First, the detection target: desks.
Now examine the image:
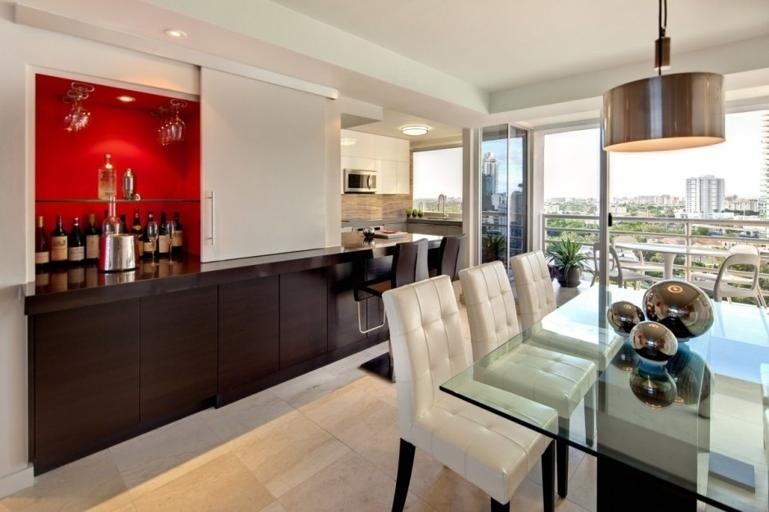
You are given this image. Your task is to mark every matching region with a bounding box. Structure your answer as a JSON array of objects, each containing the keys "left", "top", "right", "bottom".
[{"left": 438, "top": 277, "right": 768, "bottom": 511}]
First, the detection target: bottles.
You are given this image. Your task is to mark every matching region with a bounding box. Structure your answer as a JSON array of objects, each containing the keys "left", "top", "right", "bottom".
[
  {"left": 96, "top": 153, "right": 116, "bottom": 200},
  {"left": 99, "top": 195, "right": 119, "bottom": 234},
  {"left": 69, "top": 268, "right": 85, "bottom": 287},
  {"left": 87, "top": 267, "right": 98, "bottom": 286},
  {"left": 159, "top": 213, "right": 170, "bottom": 258},
  {"left": 172, "top": 261, "right": 183, "bottom": 274},
  {"left": 144, "top": 211, "right": 159, "bottom": 259},
  {"left": 158, "top": 261, "right": 170, "bottom": 277},
  {"left": 35, "top": 271, "right": 50, "bottom": 293},
  {"left": 140, "top": 261, "right": 156, "bottom": 278},
  {"left": 51, "top": 269, "right": 68, "bottom": 291},
  {"left": 170, "top": 211, "right": 184, "bottom": 259},
  {"left": 51, "top": 216, "right": 68, "bottom": 268},
  {"left": 68, "top": 217, "right": 86, "bottom": 266},
  {"left": 85, "top": 213, "right": 100, "bottom": 265},
  {"left": 132, "top": 211, "right": 144, "bottom": 260},
  {"left": 120, "top": 213, "right": 127, "bottom": 233},
  {"left": 124, "top": 169, "right": 133, "bottom": 199},
  {"left": 34, "top": 215, "right": 50, "bottom": 269}
]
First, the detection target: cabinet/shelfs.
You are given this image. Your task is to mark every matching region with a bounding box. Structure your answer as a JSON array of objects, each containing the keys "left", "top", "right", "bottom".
[
  {"left": 379, "top": 136, "right": 410, "bottom": 195},
  {"left": 339, "top": 129, "right": 380, "bottom": 162}
]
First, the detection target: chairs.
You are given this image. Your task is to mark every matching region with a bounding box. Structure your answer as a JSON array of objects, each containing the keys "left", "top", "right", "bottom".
[
  {"left": 587, "top": 222, "right": 768, "bottom": 310},
  {"left": 352, "top": 234, "right": 471, "bottom": 382},
  {"left": 510, "top": 248, "right": 634, "bottom": 446},
  {"left": 457, "top": 260, "right": 593, "bottom": 501},
  {"left": 379, "top": 272, "right": 559, "bottom": 512}
]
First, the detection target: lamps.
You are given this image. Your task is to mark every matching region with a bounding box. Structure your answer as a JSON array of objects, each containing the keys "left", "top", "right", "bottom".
[
  {"left": 599, "top": 0, "right": 726, "bottom": 155},
  {"left": 401, "top": 127, "right": 429, "bottom": 138}
]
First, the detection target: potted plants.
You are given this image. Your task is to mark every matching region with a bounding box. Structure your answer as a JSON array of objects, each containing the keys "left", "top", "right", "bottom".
[
  {"left": 548, "top": 238, "right": 585, "bottom": 288},
  {"left": 404, "top": 209, "right": 424, "bottom": 219}
]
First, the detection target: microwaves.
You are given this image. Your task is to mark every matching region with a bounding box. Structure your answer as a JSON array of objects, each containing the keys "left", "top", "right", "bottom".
[{"left": 343, "top": 169, "right": 376, "bottom": 193}]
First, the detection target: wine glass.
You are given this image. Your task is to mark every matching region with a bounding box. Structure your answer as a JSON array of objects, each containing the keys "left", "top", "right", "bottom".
[
  {"left": 64, "top": 94, "right": 91, "bottom": 132},
  {"left": 156, "top": 104, "right": 186, "bottom": 147}
]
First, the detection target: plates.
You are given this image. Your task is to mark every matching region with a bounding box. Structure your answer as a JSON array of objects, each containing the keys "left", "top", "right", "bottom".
[{"left": 384, "top": 230, "right": 399, "bottom": 234}]
